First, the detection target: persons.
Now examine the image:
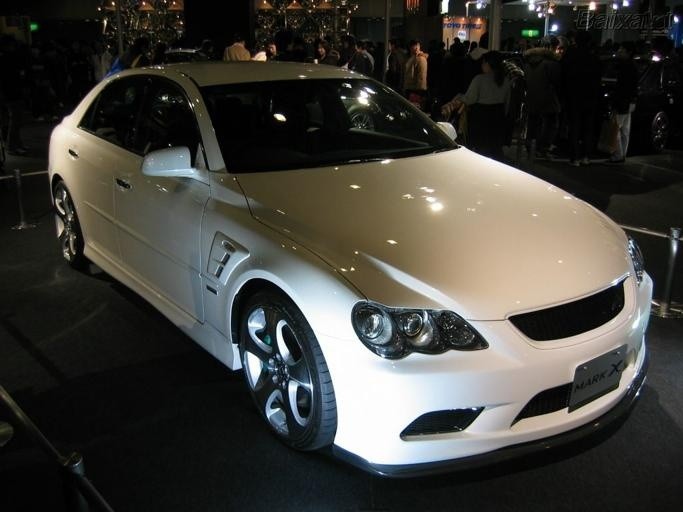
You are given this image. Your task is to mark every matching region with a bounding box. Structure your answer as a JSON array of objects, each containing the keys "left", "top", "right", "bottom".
[{"left": 1, "top": 24, "right": 642, "bottom": 167}]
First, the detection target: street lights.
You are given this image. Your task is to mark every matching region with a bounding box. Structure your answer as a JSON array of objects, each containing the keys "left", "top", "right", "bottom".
[
  {"left": 529, "top": 0, "right": 558, "bottom": 37},
  {"left": 466, "top": 0, "right": 488, "bottom": 41}
]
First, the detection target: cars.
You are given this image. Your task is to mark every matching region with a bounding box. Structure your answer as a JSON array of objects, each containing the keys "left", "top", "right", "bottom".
[
  {"left": 594, "top": 55, "right": 682, "bottom": 155},
  {"left": 48, "top": 60, "right": 655, "bottom": 480}
]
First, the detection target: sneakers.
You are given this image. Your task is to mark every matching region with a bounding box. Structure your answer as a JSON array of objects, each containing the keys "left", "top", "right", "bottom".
[{"left": 531, "top": 152, "right": 628, "bottom": 168}]
